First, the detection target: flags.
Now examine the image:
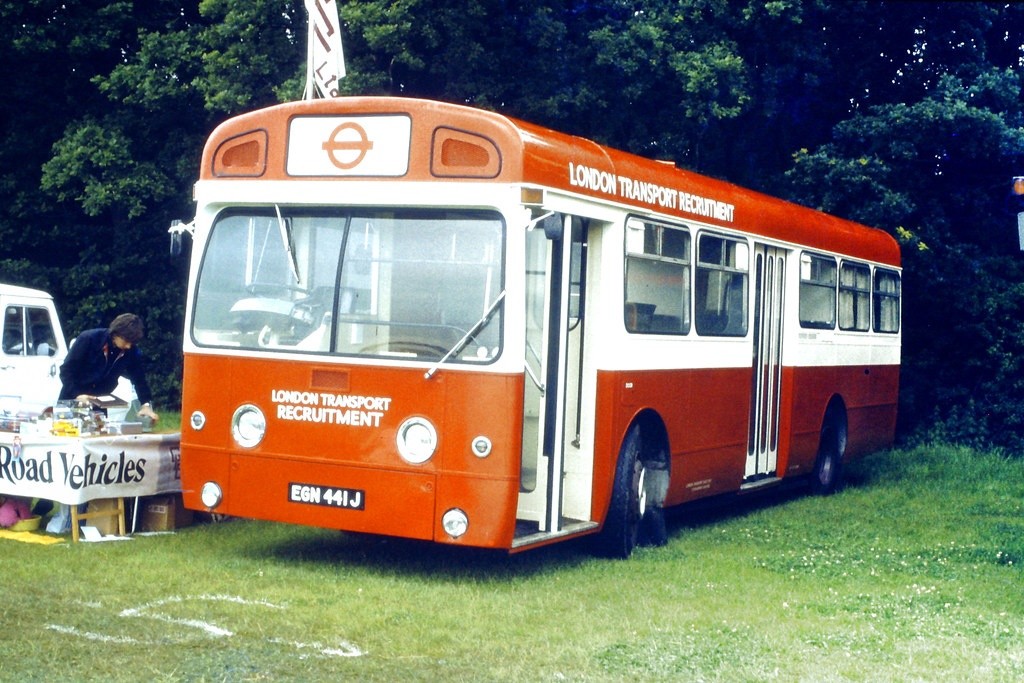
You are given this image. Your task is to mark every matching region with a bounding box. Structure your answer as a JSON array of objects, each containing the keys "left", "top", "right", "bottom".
[{"left": 304, "top": 0, "right": 347, "bottom": 101}]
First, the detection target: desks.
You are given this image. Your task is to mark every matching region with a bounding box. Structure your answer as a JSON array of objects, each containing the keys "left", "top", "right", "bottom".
[{"left": 0, "top": 431, "right": 184, "bottom": 543}]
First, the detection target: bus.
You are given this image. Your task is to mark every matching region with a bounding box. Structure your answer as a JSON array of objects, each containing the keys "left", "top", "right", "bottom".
[{"left": 170, "top": 95, "right": 903, "bottom": 560}]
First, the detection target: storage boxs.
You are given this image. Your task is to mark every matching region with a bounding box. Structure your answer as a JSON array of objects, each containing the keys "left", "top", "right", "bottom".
[{"left": 140, "top": 493, "right": 195, "bottom": 531}]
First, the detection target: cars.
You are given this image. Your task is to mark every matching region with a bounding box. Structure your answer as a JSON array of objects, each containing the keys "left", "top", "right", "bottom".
[{"left": 1, "top": 282, "right": 78, "bottom": 414}]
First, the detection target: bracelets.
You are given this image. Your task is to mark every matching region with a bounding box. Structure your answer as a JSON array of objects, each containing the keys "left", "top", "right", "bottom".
[{"left": 141, "top": 402, "right": 152, "bottom": 407}]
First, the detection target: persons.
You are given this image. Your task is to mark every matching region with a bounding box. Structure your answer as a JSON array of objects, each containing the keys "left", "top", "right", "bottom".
[{"left": 31, "top": 314, "right": 158, "bottom": 516}]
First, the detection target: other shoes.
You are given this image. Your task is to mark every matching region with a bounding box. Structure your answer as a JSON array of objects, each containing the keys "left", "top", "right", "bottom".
[{"left": 32, "top": 500, "right": 55, "bottom": 517}]
